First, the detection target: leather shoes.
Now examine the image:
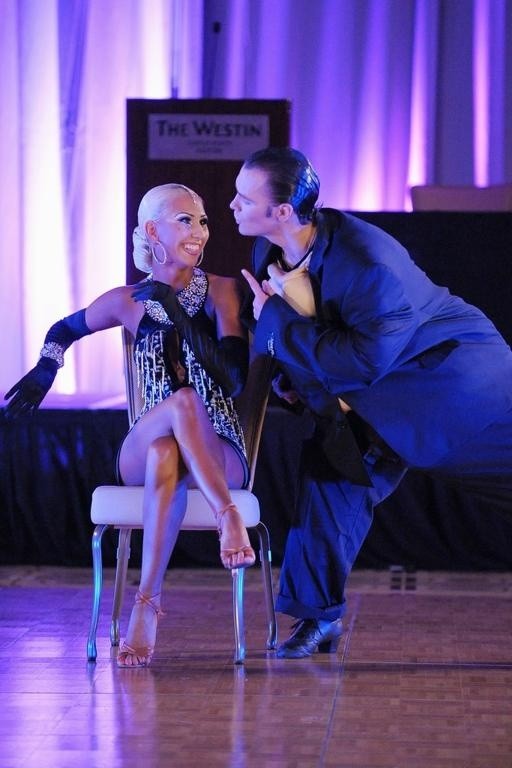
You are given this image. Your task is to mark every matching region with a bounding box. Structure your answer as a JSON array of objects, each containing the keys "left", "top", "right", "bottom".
[{"left": 276, "top": 612, "right": 344, "bottom": 660}]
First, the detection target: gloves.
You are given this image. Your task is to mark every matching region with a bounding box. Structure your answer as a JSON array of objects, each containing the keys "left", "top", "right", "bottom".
[
  {"left": 129, "top": 278, "right": 250, "bottom": 396},
  {"left": 5, "top": 308, "right": 92, "bottom": 417}
]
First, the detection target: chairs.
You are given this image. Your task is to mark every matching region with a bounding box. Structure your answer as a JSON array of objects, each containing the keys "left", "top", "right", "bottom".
[{"left": 85, "top": 324, "right": 279, "bottom": 665}]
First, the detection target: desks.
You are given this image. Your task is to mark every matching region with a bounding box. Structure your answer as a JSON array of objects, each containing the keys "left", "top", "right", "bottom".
[{"left": 1, "top": 407, "right": 294, "bottom": 567}]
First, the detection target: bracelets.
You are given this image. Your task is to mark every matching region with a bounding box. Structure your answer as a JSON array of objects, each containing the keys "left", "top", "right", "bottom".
[{"left": 39, "top": 343, "right": 65, "bottom": 367}]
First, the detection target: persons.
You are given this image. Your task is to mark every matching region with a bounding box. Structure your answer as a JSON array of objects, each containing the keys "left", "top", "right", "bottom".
[
  {"left": 6, "top": 183, "right": 255, "bottom": 669},
  {"left": 231, "top": 147, "right": 512, "bottom": 659}
]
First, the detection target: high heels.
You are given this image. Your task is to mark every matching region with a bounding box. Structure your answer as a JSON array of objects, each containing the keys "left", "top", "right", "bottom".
[
  {"left": 116, "top": 588, "right": 165, "bottom": 669},
  {"left": 214, "top": 502, "right": 256, "bottom": 576}
]
